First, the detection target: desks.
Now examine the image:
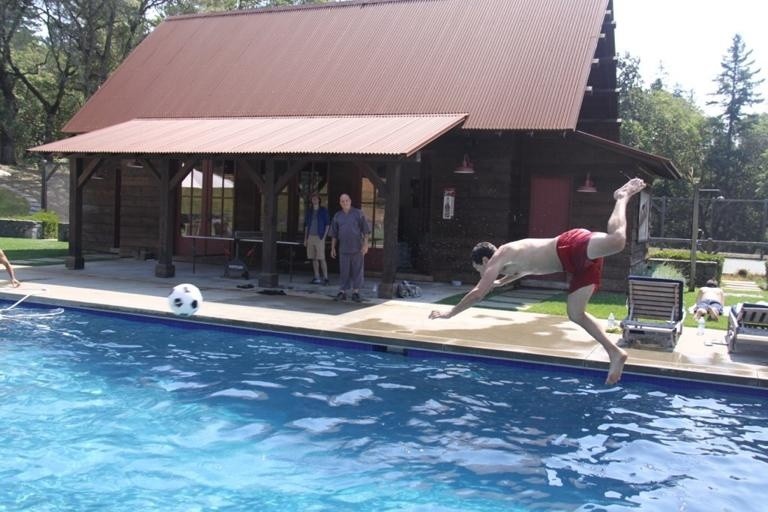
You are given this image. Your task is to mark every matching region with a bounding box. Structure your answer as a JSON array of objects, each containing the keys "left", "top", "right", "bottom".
[{"left": 184, "top": 231, "right": 304, "bottom": 283}]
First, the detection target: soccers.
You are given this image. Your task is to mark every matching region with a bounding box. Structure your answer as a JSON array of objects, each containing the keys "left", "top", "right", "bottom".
[{"left": 169, "top": 283, "right": 203, "bottom": 318}]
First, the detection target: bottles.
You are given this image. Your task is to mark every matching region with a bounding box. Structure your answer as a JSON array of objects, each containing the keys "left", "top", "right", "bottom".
[
  {"left": 607, "top": 313, "right": 616, "bottom": 329},
  {"left": 697, "top": 317, "right": 705, "bottom": 335}
]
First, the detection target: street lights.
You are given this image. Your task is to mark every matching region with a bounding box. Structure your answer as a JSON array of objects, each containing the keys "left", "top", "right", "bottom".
[{"left": 687, "top": 185, "right": 726, "bottom": 295}]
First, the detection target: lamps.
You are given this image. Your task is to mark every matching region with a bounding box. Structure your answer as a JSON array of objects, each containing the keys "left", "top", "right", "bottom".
[
  {"left": 576, "top": 171, "right": 597, "bottom": 193},
  {"left": 127, "top": 159, "right": 144, "bottom": 168},
  {"left": 453, "top": 154, "right": 474, "bottom": 174}
]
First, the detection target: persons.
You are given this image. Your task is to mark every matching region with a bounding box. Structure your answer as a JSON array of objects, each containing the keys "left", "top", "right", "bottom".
[
  {"left": 0, "top": 249, "right": 20, "bottom": 287},
  {"left": 693, "top": 279, "right": 723, "bottom": 321},
  {"left": 327, "top": 194, "right": 372, "bottom": 302},
  {"left": 304, "top": 192, "right": 330, "bottom": 286},
  {"left": 429, "top": 178, "right": 646, "bottom": 385}
]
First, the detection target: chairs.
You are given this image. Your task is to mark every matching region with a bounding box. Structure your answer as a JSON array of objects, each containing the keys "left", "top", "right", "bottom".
[
  {"left": 727, "top": 303, "right": 768, "bottom": 354},
  {"left": 620, "top": 275, "right": 686, "bottom": 347}
]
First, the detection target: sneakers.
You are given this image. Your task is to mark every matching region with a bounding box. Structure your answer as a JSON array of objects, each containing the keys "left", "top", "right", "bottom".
[
  {"left": 309, "top": 278, "right": 320, "bottom": 284},
  {"left": 334, "top": 292, "right": 347, "bottom": 300},
  {"left": 323, "top": 278, "right": 329, "bottom": 286},
  {"left": 351, "top": 293, "right": 361, "bottom": 303}
]
